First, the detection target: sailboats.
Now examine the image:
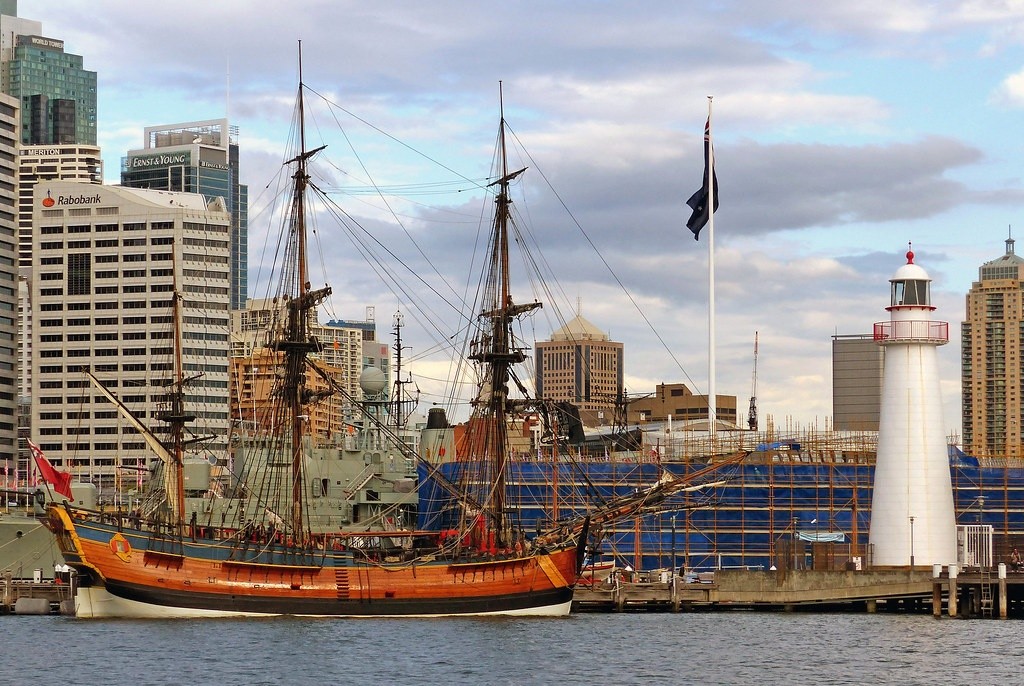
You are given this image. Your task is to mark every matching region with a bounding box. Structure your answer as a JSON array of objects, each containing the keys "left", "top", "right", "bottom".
[{"left": 23, "top": 40, "right": 752, "bottom": 618}]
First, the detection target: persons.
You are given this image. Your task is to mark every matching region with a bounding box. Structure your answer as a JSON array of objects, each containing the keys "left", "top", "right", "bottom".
[
  {"left": 128, "top": 508, "right": 141, "bottom": 530},
  {"left": 268, "top": 521, "right": 275, "bottom": 544},
  {"left": 255, "top": 521, "right": 266, "bottom": 542},
  {"left": 1010, "top": 548, "right": 1021, "bottom": 575},
  {"left": 245, "top": 519, "right": 254, "bottom": 540}
]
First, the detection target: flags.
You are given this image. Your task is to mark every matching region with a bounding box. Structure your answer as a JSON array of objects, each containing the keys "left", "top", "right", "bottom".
[
  {"left": 138, "top": 460, "right": 144, "bottom": 474},
  {"left": 5, "top": 462, "right": 8, "bottom": 483},
  {"left": 14, "top": 468, "right": 16, "bottom": 491},
  {"left": 32, "top": 466, "right": 36, "bottom": 488},
  {"left": 27, "top": 437, "right": 73, "bottom": 500},
  {"left": 138, "top": 476, "right": 142, "bottom": 487},
  {"left": 686, "top": 114, "right": 718, "bottom": 241}
]
